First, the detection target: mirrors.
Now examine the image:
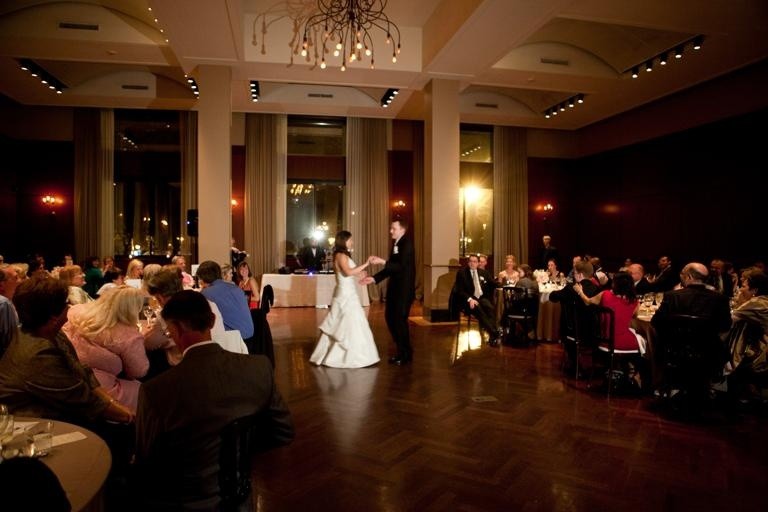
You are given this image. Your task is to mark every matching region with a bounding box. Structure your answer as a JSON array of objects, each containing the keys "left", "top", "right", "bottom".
[{"left": 286, "top": 185, "right": 345, "bottom": 254}]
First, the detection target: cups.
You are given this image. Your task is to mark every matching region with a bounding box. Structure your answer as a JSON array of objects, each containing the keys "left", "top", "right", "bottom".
[
  {"left": 636, "top": 294, "right": 662, "bottom": 315},
  {"left": 0, "top": 405, "right": 52, "bottom": 461}
]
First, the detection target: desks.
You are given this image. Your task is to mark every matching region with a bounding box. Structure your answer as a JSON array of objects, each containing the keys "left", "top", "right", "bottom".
[{"left": 258, "top": 273, "right": 373, "bottom": 312}]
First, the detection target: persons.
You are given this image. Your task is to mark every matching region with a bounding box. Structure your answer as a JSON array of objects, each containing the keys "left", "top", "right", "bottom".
[
  {"left": 447, "top": 253, "right": 767, "bottom": 440},
  {"left": 540, "top": 235, "right": 564, "bottom": 266},
  {"left": 2, "top": 238, "right": 296, "bottom": 512},
  {"left": 291, "top": 237, "right": 327, "bottom": 275},
  {"left": 359, "top": 217, "right": 416, "bottom": 367},
  {"left": 308, "top": 230, "right": 381, "bottom": 369}
]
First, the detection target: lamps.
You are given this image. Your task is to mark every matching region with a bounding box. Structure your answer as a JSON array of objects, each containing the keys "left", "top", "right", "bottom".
[
  {"left": 631, "top": 39, "right": 702, "bottom": 81},
  {"left": 287, "top": 183, "right": 314, "bottom": 206},
  {"left": 251, "top": 1, "right": 404, "bottom": 71},
  {"left": 21, "top": 57, "right": 63, "bottom": 96},
  {"left": 379, "top": 88, "right": 400, "bottom": 110},
  {"left": 41, "top": 193, "right": 65, "bottom": 215},
  {"left": 184, "top": 74, "right": 200, "bottom": 101},
  {"left": 231, "top": 200, "right": 237, "bottom": 220},
  {"left": 540, "top": 203, "right": 553, "bottom": 223},
  {"left": 392, "top": 200, "right": 406, "bottom": 223},
  {"left": 541, "top": 94, "right": 587, "bottom": 121},
  {"left": 248, "top": 80, "right": 261, "bottom": 105}
]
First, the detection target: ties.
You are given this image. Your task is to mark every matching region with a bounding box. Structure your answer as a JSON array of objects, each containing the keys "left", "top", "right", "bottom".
[{"left": 473, "top": 270, "right": 480, "bottom": 299}]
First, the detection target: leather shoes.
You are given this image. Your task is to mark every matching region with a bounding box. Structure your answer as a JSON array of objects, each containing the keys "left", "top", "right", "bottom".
[
  {"left": 490, "top": 338, "right": 497, "bottom": 344},
  {"left": 493, "top": 331, "right": 503, "bottom": 338},
  {"left": 396, "top": 357, "right": 412, "bottom": 365},
  {"left": 390, "top": 355, "right": 402, "bottom": 362}
]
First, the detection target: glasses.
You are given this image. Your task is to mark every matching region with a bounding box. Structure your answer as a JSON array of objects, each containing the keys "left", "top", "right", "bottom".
[
  {"left": 468, "top": 260, "right": 478, "bottom": 264},
  {"left": 162, "top": 320, "right": 177, "bottom": 339},
  {"left": 64, "top": 298, "right": 72, "bottom": 305}
]
